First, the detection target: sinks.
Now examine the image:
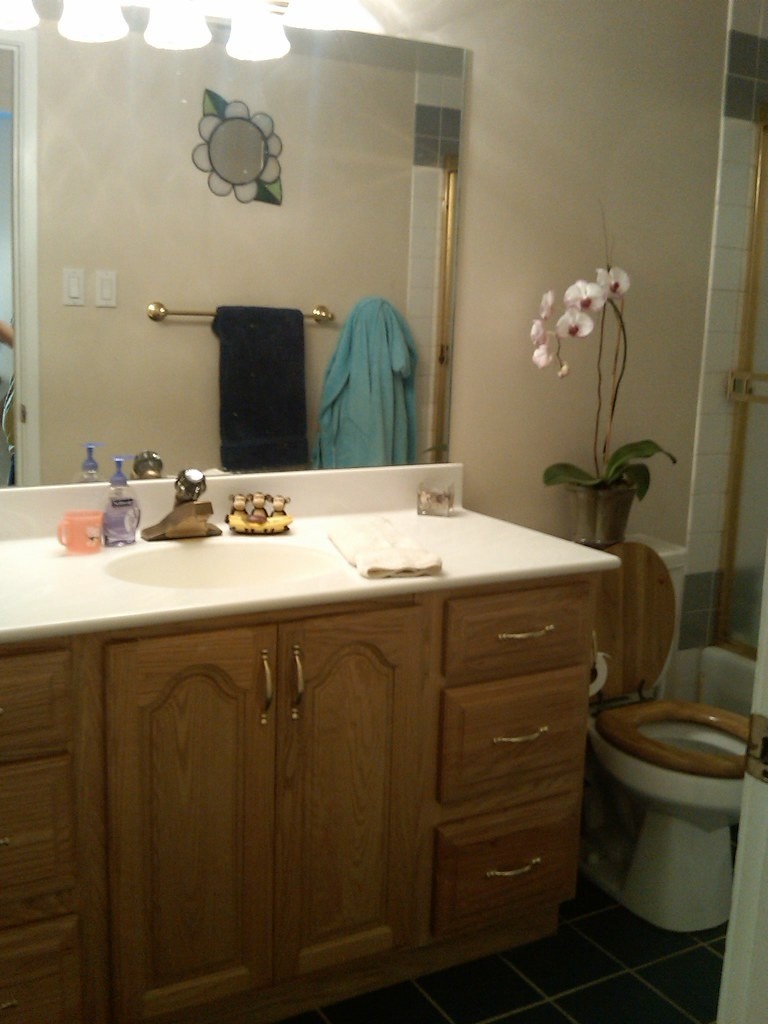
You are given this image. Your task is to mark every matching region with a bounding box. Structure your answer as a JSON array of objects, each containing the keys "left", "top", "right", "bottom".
[{"left": 101, "top": 534, "right": 334, "bottom": 593}]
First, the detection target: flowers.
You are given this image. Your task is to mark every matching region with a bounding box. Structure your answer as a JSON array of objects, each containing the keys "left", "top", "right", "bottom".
[{"left": 522, "top": 268, "right": 679, "bottom": 501}]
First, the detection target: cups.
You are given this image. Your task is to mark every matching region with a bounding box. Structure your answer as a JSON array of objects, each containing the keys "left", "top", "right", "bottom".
[
  {"left": 416, "top": 478, "right": 454, "bottom": 516},
  {"left": 57, "top": 510, "right": 103, "bottom": 554}
]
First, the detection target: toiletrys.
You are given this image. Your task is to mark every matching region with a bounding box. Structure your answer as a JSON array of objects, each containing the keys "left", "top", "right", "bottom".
[
  {"left": 101, "top": 456, "right": 140, "bottom": 547},
  {"left": 74, "top": 444, "right": 105, "bottom": 483}
]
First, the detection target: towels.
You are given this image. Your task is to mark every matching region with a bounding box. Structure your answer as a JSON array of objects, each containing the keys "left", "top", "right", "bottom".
[
  {"left": 327, "top": 514, "right": 443, "bottom": 581},
  {"left": 210, "top": 305, "right": 310, "bottom": 474},
  {"left": 311, "top": 296, "right": 419, "bottom": 472}
]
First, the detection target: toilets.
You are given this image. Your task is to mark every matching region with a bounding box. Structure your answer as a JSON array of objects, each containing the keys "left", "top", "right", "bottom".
[{"left": 569, "top": 530, "right": 750, "bottom": 932}]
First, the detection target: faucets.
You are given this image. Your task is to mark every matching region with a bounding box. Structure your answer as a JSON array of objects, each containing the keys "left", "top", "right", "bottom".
[{"left": 141, "top": 469, "right": 223, "bottom": 541}]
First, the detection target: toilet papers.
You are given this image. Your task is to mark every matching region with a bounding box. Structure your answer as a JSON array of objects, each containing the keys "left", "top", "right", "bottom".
[{"left": 590, "top": 651, "right": 611, "bottom": 697}]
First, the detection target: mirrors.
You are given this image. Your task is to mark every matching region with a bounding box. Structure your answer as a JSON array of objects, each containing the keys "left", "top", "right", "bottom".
[{"left": 0, "top": 1, "right": 469, "bottom": 489}]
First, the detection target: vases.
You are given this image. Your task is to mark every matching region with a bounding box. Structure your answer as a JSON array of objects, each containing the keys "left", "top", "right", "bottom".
[{"left": 563, "top": 483, "right": 632, "bottom": 542}]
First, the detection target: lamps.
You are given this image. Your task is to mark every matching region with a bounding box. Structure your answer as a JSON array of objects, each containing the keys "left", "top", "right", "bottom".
[
  {"left": 58, "top": 1, "right": 128, "bottom": 45},
  {"left": 145, "top": 0, "right": 211, "bottom": 50},
  {"left": 0, "top": 0, "right": 40, "bottom": 32},
  {"left": 226, "top": 1, "right": 292, "bottom": 62}
]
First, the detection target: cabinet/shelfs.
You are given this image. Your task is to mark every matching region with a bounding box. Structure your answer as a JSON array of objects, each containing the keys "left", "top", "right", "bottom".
[
  {"left": 0, "top": 645, "right": 84, "bottom": 1024},
  {"left": 101, "top": 600, "right": 425, "bottom": 1021},
  {"left": 430, "top": 584, "right": 578, "bottom": 946}
]
